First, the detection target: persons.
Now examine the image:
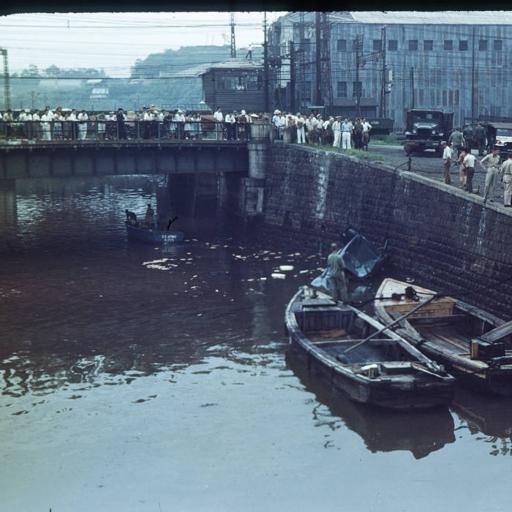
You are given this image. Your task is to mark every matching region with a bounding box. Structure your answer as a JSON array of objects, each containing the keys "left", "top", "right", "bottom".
[
  {"left": 272, "top": 109, "right": 372, "bottom": 152},
  {"left": 461, "top": 149, "right": 481, "bottom": 194},
  {"left": 473, "top": 121, "right": 487, "bottom": 155},
  {"left": 326, "top": 242, "right": 351, "bottom": 305},
  {"left": 145, "top": 202, "right": 154, "bottom": 229},
  {"left": 213, "top": 107, "right": 252, "bottom": 141},
  {"left": 124, "top": 208, "right": 137, "bottom": 226},
  {"left": 498, "top": 153, "right": 512, "bottom": 207},
  {"left": 457, "top": 145, "right": 467, "bottom": 184},
  {"left": 478, "top": 148, "right": 503, "bottom": 204},
  {"left": 462, "top": 122, "right": 474, "bottom": 153},
  {"left": 447, "top": 126, "right": 467, "bottom": 168},
  {"left": 440, "top": 140, "right": 454, "bottom": 186},
  {"left": 2, "top": 104, "right": 203, "bottom": 141}
]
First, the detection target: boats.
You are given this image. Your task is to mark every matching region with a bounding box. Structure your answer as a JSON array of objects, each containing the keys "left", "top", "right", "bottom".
[
  {"left": 284, "top": 277, "right": 512, "bottom": 414},
  {"left": 125, "top": 221, "right": 184, "bottom": 246}
]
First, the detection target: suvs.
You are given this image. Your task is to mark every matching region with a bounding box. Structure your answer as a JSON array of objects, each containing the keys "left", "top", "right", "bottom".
[{"left": 484, "top": 121, "right": 512, "bottom": 156}]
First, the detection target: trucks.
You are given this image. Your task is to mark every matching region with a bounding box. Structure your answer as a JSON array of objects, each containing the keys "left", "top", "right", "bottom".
[{"left": 404, "top": 109, "right": 454, "bottom": 153}]
